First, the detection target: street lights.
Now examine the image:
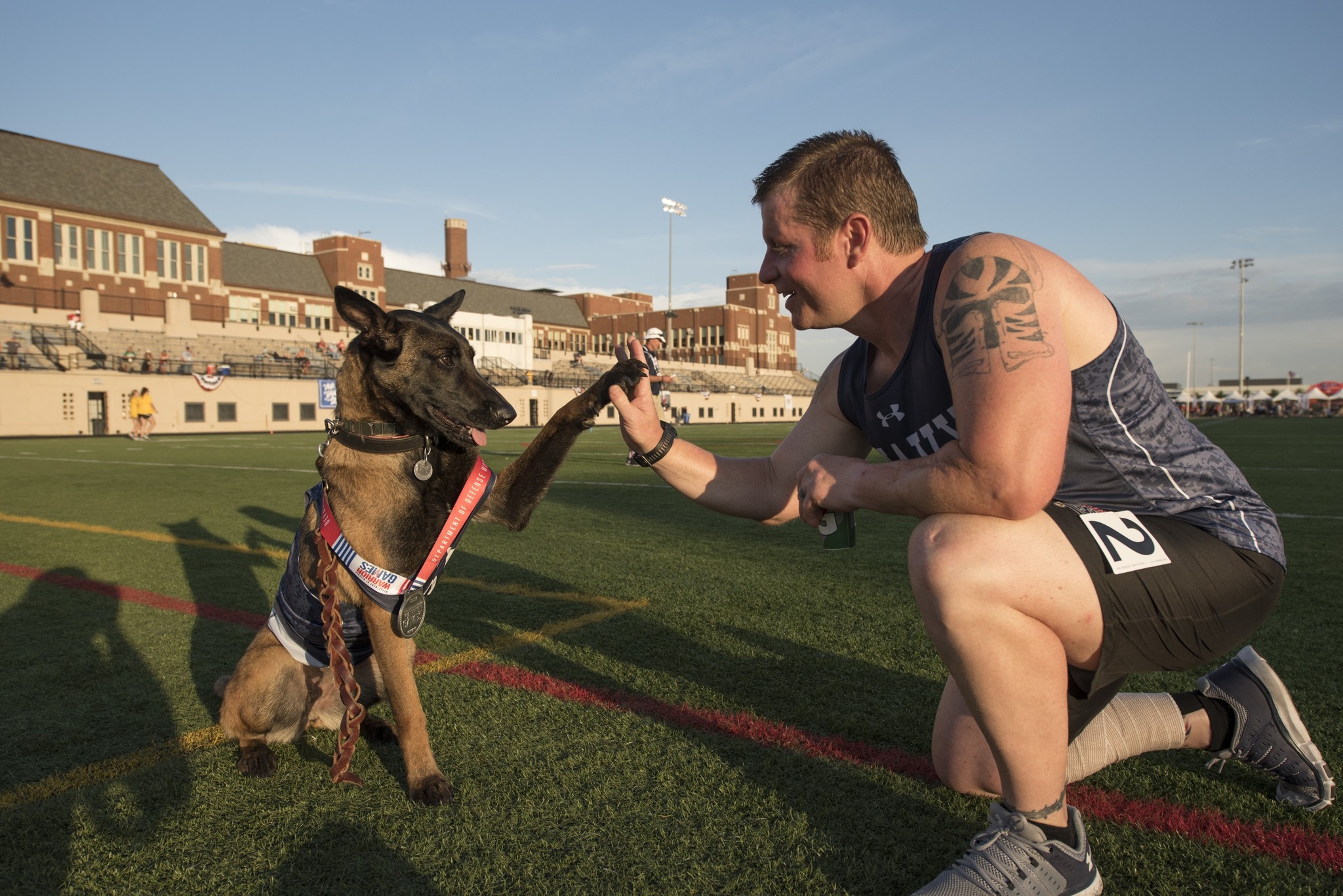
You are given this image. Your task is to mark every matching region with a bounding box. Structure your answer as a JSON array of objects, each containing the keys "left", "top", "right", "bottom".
[
  {"left": 1186, "top": 321, "right": 1205, "bottom": 396},
  {"left": 662, "top": 198, "right": 688, "bottom": 312},
  {"left": 1229, "top": 257, "right": 1255, "bottom": 398}
]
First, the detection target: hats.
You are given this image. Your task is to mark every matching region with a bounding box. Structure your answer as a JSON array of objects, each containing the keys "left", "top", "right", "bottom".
[
  {"left": 75, "top": 309, "right": 80, "bottom": 314},
  {"left": 643, "top": 327, "right": 667, "bottom": 343}
]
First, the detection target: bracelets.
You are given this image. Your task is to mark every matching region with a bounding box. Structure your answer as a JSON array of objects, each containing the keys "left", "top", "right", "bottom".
[{"left": 625, "top": 420, "right": 677, "bottom": 467}]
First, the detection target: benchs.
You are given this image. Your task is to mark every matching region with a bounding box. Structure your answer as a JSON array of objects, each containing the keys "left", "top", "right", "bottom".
[{"left": 0, "top": 320, "right": 815, "bottom": 396}]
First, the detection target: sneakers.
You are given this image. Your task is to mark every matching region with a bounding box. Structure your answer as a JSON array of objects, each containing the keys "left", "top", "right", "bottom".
[
  {"left": 1194, "top": 643, "right": 1334, "bottom": 814},
  {"left": 910, "top": 796, "right": 1103, "bottom": 896}
]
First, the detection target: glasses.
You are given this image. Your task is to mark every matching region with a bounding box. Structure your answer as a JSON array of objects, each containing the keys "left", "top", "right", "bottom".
[{"left": 649, "top": 333, "right": 664, "bottom": 337}]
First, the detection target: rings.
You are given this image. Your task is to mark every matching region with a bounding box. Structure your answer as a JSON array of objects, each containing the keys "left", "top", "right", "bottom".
[{"left": 798, "top": 488, "right": 805, "bottom": 500}]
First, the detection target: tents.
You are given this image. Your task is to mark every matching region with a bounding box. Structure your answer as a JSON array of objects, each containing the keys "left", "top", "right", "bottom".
[
  {"left": 1247, "top": 390, "right": 1272, "bottom": 402},
  {"left": 1197, "top": 391, "right": 1221, "bottom": 403},
  {"left": 1222, "top": 391, "right": 1245, "bottom": 402},
  {"left": 1175, "top": 390, "right": 1194, "bottom": 403},
  {"left": 1330, "top": 388, "right": 1343, "bottom": 401},
  {"left": 1272, "top": 388, "right": 1299, "bottom": 403},
  {"left": 1304, "top": 387, "right": 1330, "bottom": 401}
]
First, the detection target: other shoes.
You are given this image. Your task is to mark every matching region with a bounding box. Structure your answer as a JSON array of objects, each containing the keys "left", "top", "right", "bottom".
[
  {"left": 129, "top": 432, "right": 140, "bottom": 440},
  {"left": 625, "top": 457, "right": 640, "bottom": 466},
  {"left": 138, "top": 435, "right": 152, "bottom": 441}
]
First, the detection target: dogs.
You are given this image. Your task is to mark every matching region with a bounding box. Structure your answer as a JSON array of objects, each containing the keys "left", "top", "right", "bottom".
[{"left": 213, "top": 286, "right": 651, "bottom": 803}]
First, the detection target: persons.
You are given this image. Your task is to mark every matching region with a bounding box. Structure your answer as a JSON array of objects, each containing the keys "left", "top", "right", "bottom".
[
  {"left": 609, "top": 129, "right": 1337, "bottom": 896},
  {"left": 136, "top": 388, "right": 158, "bottom": 441},
  {"left": 68, "top": 310, "right": 84, "bottom": 331},
  {"left": 144, "top": 350, "right": 155, "bottom": 373},
  {"left": 576, "top": 354, "right": 583, "bottom": 365},
  {"left": 130, "top": 390, "right": 141, "bottom": 442},
  {"left": 181, "top": 347, "right": 196, "bottom": 374},
  {"left": 6, "top": 337, "right": 27, "bottom": 370},
  {"left": 263, "top": 346, "right": 310, "bottom": 375},
  {"left": 123, "top": 347, "right": 140, "bottom": 373},
  {"left": 159, "top": 351, "right": 171, "bottom": 373},
  {"left": 318, "top": 339, "right": 345, "bottom": 362}
]
